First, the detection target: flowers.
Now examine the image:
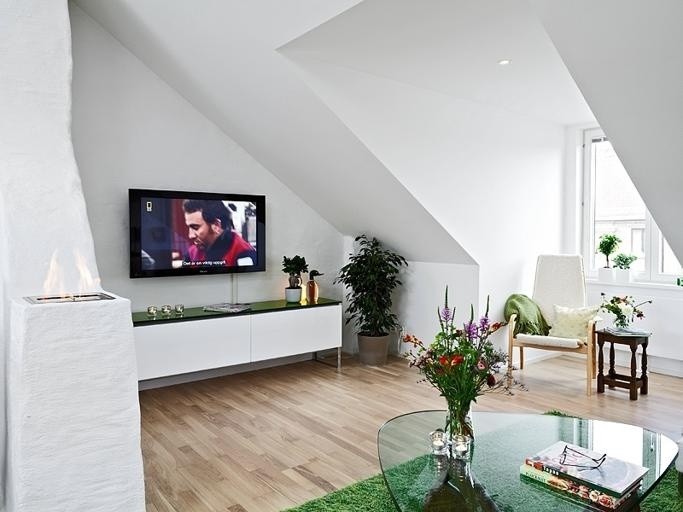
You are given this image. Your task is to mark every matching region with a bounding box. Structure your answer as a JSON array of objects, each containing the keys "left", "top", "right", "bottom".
[
  {"left": 401, "top": 284, "right": 529, "bottom": 431},
  {"left": 600, "top": 292, "right": 652, "bottom": 327}
]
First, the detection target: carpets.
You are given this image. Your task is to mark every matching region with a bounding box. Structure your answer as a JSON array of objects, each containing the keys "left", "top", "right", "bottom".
[{"left": 277, "top": 409, "right": 682, "bottom": 511}]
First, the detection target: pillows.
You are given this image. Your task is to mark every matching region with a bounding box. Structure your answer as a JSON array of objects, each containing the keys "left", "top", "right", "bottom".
[{"left": 546, "top": 304, "right": 599, "bottom": 345}]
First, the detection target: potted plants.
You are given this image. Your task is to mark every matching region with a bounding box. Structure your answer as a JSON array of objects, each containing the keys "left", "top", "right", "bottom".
[
  {"left": 333, "top": 233, "right": 409, "bottom": 366},
  {"left": 281, "top": 255, "right": 310, "bottom": 302},
  {"left": 596, "top": 232, "right": 623, "bottom": 283},
  {"left": 611, "top": 253, "right": 638, "bottom": 285}
]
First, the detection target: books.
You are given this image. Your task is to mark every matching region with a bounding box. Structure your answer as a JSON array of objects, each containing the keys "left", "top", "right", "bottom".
[
  {"left": 202, "top": 301, "right": 250, "bottom": 313},
  {"left": 520, "top": 464, "right": 644, "bottom": 510},
  {"left": 526, "top": 440, "right": 651, "bottom": 498}
]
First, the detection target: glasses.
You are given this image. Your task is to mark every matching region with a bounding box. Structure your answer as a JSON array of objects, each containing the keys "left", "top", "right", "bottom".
[{"left": 557, "top": 443, "right": 607, "bottom": 470}]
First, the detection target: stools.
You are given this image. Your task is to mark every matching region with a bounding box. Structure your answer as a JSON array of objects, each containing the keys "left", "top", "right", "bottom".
[{"left": 594, "top": 328, "right": 653, "bottom": 401}]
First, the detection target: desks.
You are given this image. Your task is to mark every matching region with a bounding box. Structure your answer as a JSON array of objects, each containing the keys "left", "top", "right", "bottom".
[{"left": 376, "top": 409, "right": 680, "bottom": 512}]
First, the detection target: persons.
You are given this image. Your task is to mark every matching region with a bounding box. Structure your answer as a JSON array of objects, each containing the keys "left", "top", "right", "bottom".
[{"left": 183, "top": 199, "right": 257, "bottom": 264}]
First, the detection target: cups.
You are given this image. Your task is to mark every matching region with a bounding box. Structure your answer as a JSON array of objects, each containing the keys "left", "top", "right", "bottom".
[
  {"left": 452, "top": 434, "right": 470, "bottom": 459},
  {"left": 148, "top": 306, "right": 158, "bottom": 318},
  {"left": 176, "top": 304, "right": 185, "bottom": 316},
  {"left": 427, "top": 431, "right": 448, "bottom": 455},
  {"left": 162, "top": 305, "right": 171, "bottom": 316}
]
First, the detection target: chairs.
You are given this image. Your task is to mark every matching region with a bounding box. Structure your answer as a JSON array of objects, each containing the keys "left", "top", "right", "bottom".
[{"left": 506, "top": 252, "right": 598, "bottom": 398}]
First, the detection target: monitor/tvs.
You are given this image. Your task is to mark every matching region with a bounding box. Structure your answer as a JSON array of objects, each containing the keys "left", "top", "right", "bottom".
[{"left": 128, "top": 188, "right": 266, "bottom": 278}]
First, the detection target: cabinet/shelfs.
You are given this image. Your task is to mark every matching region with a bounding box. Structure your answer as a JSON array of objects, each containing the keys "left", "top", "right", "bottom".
[{"left": 132, "top": 296, "right": 342, "bottom": 382}]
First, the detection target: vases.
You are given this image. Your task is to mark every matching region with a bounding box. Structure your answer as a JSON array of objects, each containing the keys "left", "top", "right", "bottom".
[{"left": 446, "top": 422, "right": 474, "bottom": 463}]
